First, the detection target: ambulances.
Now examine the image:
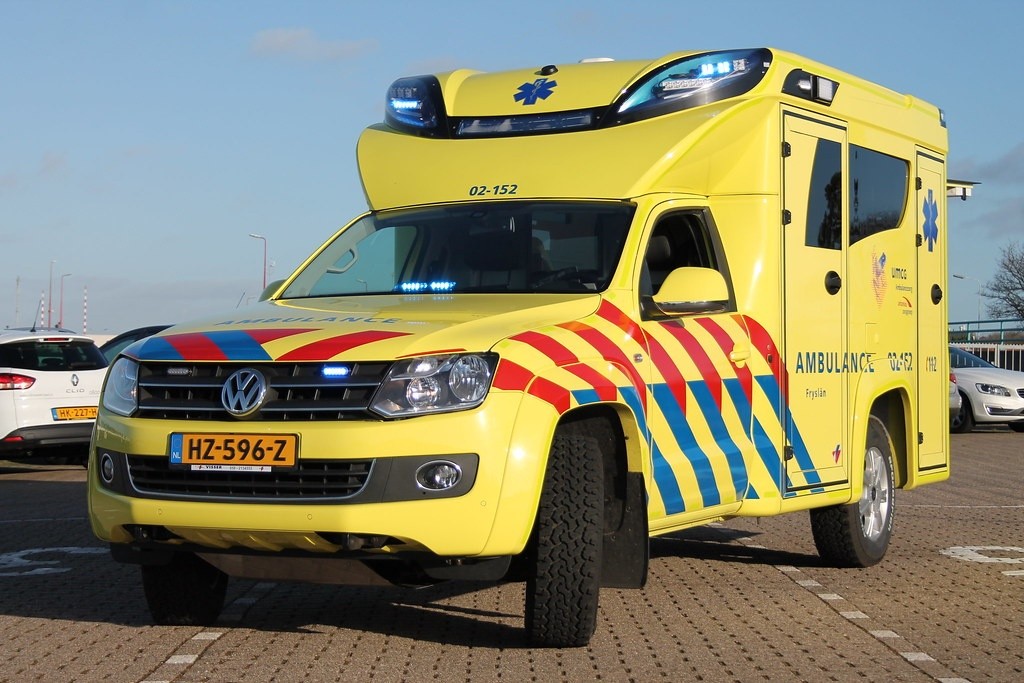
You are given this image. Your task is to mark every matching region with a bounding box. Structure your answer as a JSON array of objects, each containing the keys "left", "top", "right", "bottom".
[{"left": 90, "top": 46, "right": 979, "bottom": 648}]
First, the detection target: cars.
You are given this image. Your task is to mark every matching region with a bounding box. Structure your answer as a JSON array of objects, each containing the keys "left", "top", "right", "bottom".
[
  {"left": 98, "top": 325, "right": 169, "bottom": 366},
  {"left": 0, "top": 329, "right": 110, "bottom": 462},
  {"left": 948, "top": 346, "right": 1023, "bottom": 433}
]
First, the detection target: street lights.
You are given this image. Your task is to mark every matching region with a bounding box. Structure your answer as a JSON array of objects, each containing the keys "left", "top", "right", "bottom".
[
  {"left": 249, "top": 233, "right": 267, "bottom": 291},
  {"left": 952, "top": 273, "right": 982, "bottom": 341},
  {"left": 48, "top": 259, "right": 56, "bottom": 329},
  {"left": 60, "top": 273, "right": 73, "bottom": 328}
]
